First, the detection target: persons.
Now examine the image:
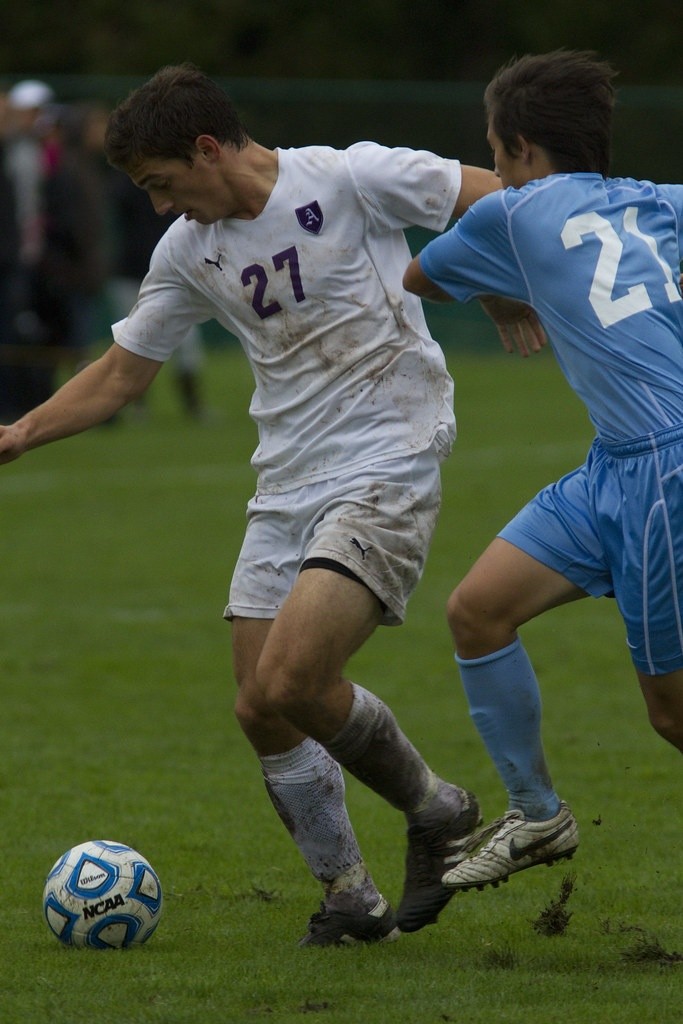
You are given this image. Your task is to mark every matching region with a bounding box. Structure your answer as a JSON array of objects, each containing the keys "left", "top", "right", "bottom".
[
  {"left": 0, "top": 69, "right": 506, "bottom": 945},
  {"left": 404, "top": 56, "right": 683, "bottom": 890},
  {"left": 0, "top": 82, "right": 205, "bottom": 422}
]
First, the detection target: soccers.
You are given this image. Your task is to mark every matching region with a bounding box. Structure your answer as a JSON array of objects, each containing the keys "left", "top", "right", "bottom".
[{"left": 42, "top": 841, "right": 163, "bottom": 950}]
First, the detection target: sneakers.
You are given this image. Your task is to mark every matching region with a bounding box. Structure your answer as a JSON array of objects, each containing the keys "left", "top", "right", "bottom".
[
  {"left": 441, "top": 796, "right": 580, "bottom": 893},
  {"left": 294, "top": 896, "right": 403, "bottom": 949},
  {"left": 397, "top": 791, "right": 484, "bottom": 934}
]
